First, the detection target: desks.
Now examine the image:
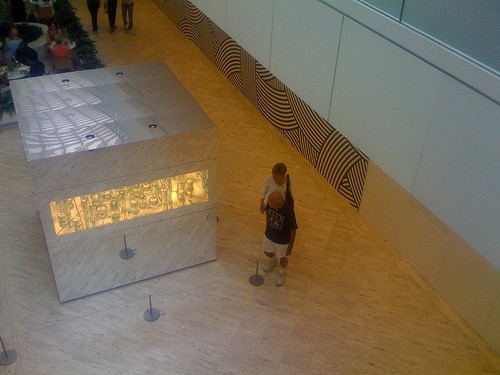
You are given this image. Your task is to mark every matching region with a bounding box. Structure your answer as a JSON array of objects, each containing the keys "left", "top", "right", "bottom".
[
  {"left": 49, "top": 40, "right": 76, "bottom": 49},
  {"left": 0, "top": 61, "right": 30, "bottom": 80}
]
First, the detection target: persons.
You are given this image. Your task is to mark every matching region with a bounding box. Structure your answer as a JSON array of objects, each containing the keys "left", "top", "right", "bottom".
[
  {"left": 46, "top": 20, "right": 63, "bottom": 57},
  {"left": 260, "top": 163, "right": 294, "bottom": 215},
  {"left": 0, "top": 27, "right": 45, "bottom": 85},
  {"left": 262, "top": 191, "right": 299, "bottom": 286},
  {"left": 0, "top": 0, "right": 57, "bottom": 21},
  {"left": 86, "top": 0, "right": 100, "bottom": 34},
  {"left": 104, "top": 0, "right": 117, "bottom": 33},
  {"left": 50, "top": 34, "right": 70, "bottom": 65},
  {"left": 121, "top": 0, "right": 135, "bottom": 35}
]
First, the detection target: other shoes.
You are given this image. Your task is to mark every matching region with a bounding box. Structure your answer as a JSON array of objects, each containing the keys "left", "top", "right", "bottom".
[
  {"left": 276, "top": 272, "right": 286, "bottom": 286},
  {"left": 263, "top": 259, "right": 276, "bottom": 272}
]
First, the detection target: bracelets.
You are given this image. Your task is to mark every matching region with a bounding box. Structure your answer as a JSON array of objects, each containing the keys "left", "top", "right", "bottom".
[{"left": 288, "top": 246, "right": 293, "bottom": 248}]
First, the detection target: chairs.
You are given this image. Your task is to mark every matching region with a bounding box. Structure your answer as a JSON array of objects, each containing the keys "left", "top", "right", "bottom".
[
  {"left": 54, "top": 55, "right": 74, "bottom": 73},
  {"left": 38, "top": 6, "right": 57, "bottom": 28}
]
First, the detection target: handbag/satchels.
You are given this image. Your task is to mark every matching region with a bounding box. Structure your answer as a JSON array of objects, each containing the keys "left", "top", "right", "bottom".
[{"left": 286, "top": 174, "right": 294, "bottom": 210}]
